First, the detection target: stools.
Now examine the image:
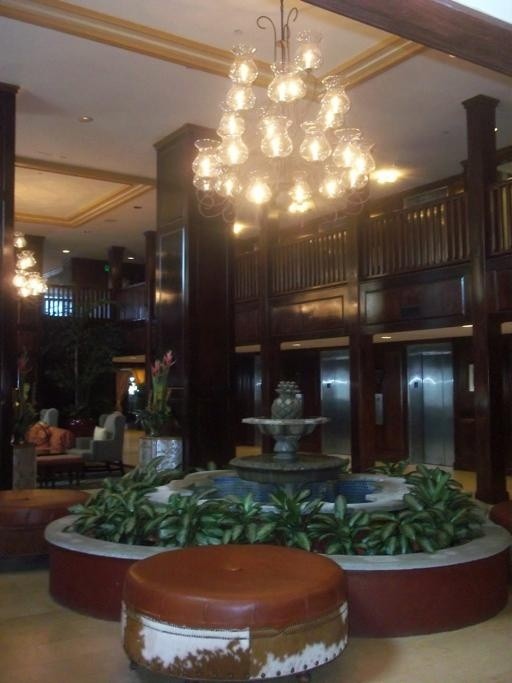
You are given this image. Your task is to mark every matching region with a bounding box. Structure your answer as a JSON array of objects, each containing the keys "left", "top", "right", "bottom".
[{"left": 122, "top": 544, "right": 351, "bottom": 681}]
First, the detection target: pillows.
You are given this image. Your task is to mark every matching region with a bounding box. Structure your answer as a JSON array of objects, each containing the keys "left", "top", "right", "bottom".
[{"left": 91, "top": 425, "right": 109, "bottom": 442}]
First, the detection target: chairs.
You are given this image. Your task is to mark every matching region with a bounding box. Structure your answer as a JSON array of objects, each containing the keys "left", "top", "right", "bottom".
[{"left": 74, "top": 411, "right": 128, "bottom": 476}]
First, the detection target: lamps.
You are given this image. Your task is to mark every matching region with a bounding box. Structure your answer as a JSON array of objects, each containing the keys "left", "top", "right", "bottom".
[
  {"left": 13, "top": 231, "right": 48, "bottom": 297},
  {"left": 190, "top": 0, "right": 374, "bottom": 237}
]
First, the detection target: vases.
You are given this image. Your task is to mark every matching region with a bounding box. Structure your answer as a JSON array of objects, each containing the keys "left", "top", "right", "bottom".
[
  {"left": 140, "top": 436, "right": 184, "bottom": 476},
  {"left": 12, "top": 445, "right": 38, "bottom": 491}
]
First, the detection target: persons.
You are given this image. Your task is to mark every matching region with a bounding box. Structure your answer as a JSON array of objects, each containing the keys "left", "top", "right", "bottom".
[
  {"left": 27, "top": 408, "right": 73, "bottom": 452},
  {"left": 128, "top": 382, "right": 139, "bottom": 414}
]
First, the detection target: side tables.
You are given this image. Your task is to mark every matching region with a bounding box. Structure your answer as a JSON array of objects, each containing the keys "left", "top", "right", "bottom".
[{"left": 37, "top": 453, "right": 80, "bottom": 488}]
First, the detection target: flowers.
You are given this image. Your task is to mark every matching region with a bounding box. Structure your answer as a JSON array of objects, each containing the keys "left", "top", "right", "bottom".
[
  {"left": 11, "top": 347, "right": 38, "bottom": 446},
  {"left": 127, "top": 347, "right": 176, "bottom": 435}
]
